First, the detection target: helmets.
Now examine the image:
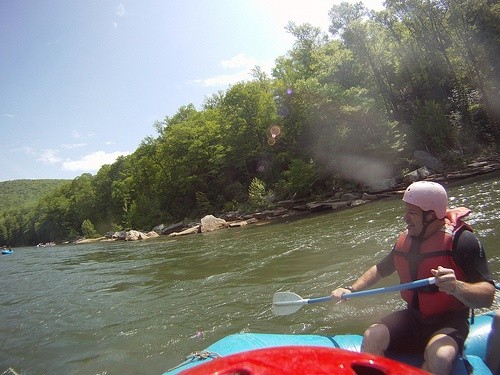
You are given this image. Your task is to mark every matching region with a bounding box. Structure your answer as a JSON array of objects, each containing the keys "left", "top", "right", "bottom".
[{"left": 402, "top": 181, "right": 448, "bottom": 220}]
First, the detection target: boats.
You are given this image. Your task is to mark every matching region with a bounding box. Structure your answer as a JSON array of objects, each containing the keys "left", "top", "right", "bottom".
[
  {"left": 159, "top": 274, "right": 499, "bottom": 375},
  {"left": 1, "top": 249, "right": 13, "bottom": 255}
]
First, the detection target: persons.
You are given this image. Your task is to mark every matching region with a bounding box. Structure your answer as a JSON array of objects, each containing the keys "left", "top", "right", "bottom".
[{"left": 331, "top": 180, "right": 495, "bottom": 374}]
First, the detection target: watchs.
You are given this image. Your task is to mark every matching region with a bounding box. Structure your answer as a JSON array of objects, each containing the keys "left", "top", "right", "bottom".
[{"left": 345, "top": 286, "right": 355, "bottom": 293}]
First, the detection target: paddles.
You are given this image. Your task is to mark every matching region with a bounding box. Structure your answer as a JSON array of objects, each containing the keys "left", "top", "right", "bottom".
[{"left": 273, "top": 277, "right": 436, "bottom": 316}]
